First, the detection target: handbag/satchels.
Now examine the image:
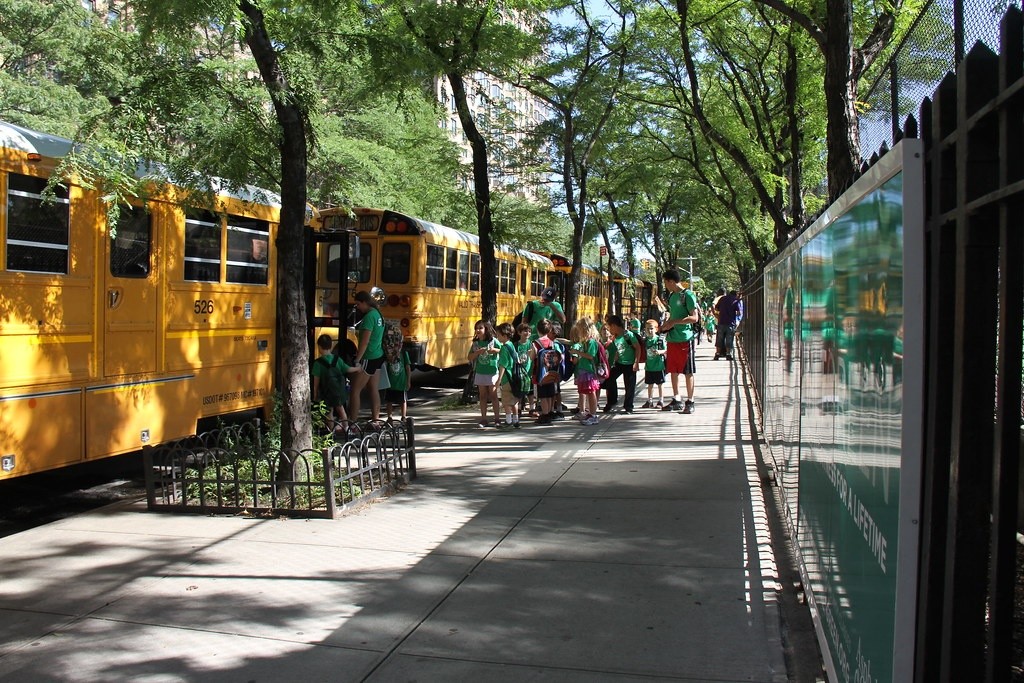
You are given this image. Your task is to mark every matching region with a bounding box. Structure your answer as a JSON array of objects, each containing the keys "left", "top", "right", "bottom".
[{"left": 510, "top": 300, "right": 534, "bottom": 342}]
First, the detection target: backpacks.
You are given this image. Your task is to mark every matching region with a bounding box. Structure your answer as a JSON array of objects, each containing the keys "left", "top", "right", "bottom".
[
  {"left": 584, "top": 339, "right": 610, "bottom": 380},
  {"left": 535, "top": 340, "right": 564, "bottom": 385},
  {"left": 683, "top": 290, "right": 706, "bottom": 345},
  {"left": 625, "top": 329, "right": 646, "bottom": 363},
  {"left": 316, "top": 355, "right": 348, "bottom": 408},
  {"left": 382, "top": 323, "right": 404, "bottom": 364},
  {"left": 501, "top": 344, "right": 534, "bottom": 398},
  {"left": 559, "top": 342, "right": 577, "bottom": 382}
]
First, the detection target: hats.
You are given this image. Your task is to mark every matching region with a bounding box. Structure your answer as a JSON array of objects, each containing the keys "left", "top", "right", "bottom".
[{"left": 541, "top": 287, "right": 556, "bottom": 302}]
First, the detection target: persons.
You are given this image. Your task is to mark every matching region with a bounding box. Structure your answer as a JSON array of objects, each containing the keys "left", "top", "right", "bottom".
[
  {"left": 495, "top": 323, "right": 522, "bottom": 429},
  {"left": 468, "top": 320, "right": 503, "bottom": 428},
  {"left": 385, "top": 348, "right": 411, "bottom": 426},
  {"left": 312, "top": 335, "right": 362, "bottom": 435},
  {"left": 662, "top": 269, "right": 699, "bottom": 414},
  {"left": 514, "top": 286, "right": 670, "bottom": 425},
  {"left": 349, "top": 290, "right": 385, "bottom": 433},
  {"left": 700, "top": 286, "right": 741, "bottom": 360}
]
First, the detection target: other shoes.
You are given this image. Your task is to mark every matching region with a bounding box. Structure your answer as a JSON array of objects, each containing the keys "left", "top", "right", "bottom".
[
  {"left": 494, "top": 420, "right": 500, "bottom": 428},
  {"left": 513, "top": 422, "right": 523, "bottom": 429},
  {"left": 497, "top": 422, "right": 513, "bottom": 430},
  {"left": 476, "top": 420, "right": 488, "bottom": 428},
  {"left": 519, "top": 403, "right": 635, "bottom": 430},
  {"left": 713, "top": 353, "right": 719, "bottom": 360},
  {"left": 726, "top": 355, "right": 733, "bottom": 360},
  {"left": 361, "top": 422, "right": 381, "bottom": 433},
  {"left": 342, "top": 424, "right": 359, "bottom": 435},
  {"left": 386, "top": 419, "right": 394, "bottom": 427},
  {"left": 401, "top": 417, "right": 407, "bottom": 426}
]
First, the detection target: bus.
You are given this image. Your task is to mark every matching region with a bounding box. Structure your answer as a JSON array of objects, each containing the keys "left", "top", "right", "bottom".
[
  {"left": 311, "top": 206, "right": 659, "bottom": 401},
  {"left": 1, "top": 121, "right": 322, "bottom": 485}
]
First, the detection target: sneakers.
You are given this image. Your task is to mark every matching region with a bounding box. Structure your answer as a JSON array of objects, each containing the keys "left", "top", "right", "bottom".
[
  {"left": 661, "top": 397, "right": 683, "bottom": 411},
  {"left": 642, "top": 401, "right": 653, "bottom": 408},
  {"left": 683, "top": 399, "right": 696, "bottom": 414},
  {"left": 655, "top": 401, "right": 664, "bottom": 409}
]
